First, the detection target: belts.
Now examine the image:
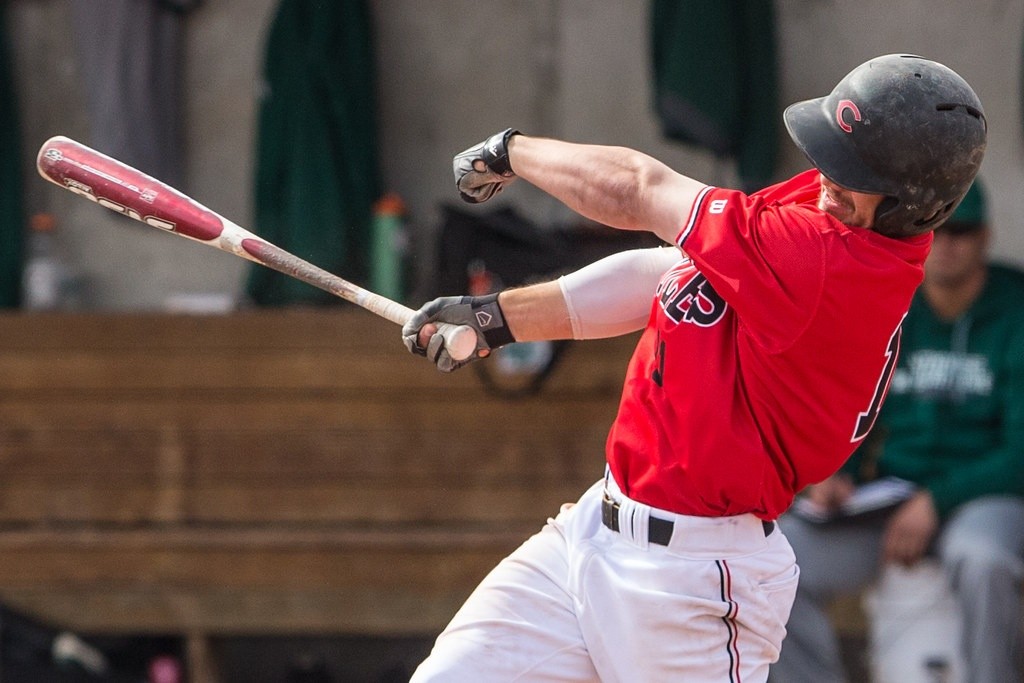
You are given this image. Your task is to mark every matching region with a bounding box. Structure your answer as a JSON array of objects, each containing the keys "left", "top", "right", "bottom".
[{"left": 599, "top": 493, "right": 776, "bottom": 546}]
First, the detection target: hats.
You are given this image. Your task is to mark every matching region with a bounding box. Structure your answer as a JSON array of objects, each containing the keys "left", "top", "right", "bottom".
[{"left": 948, "top": 178, "right": 987, "bottom": 226}]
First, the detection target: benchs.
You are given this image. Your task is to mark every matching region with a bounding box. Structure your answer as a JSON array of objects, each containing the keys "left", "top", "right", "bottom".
[{"left": 0, "top": 318, "right": 877, "bottom": 683}]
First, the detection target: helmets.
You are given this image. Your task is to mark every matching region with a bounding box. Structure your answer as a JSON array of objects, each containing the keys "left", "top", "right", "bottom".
[{"left": 781, "top": 51, "right": 987, "bottom": 239}]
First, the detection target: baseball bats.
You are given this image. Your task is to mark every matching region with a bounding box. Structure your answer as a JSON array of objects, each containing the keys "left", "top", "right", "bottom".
[{"left": 34, "top": 135, "right": 478, "bottom": 361}]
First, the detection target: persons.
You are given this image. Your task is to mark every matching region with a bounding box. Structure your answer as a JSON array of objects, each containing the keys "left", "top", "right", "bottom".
[
  {"left": 410, "top": 54, "right": 988, "bottom": 683},
  {"left": 765, "top": 170, "right": 1024, "bottom": 683}
]
